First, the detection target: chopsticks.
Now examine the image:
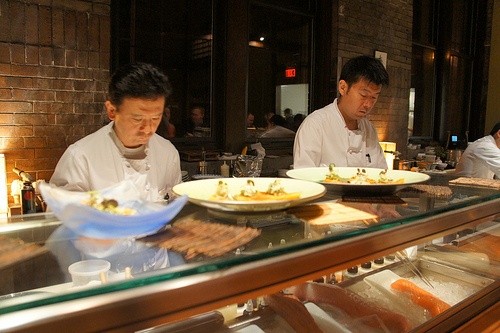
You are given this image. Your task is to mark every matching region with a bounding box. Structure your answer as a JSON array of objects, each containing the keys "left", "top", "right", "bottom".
[{"left": 396, "top": 250, "right": 434, "bottom": 288}]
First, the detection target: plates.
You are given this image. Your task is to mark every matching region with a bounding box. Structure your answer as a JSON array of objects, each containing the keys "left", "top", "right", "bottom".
[
  {"left": 192, "top": 174, "right": 222, "bottom": 179},
  {"left": 285, "top": 167, "right": 430, "bottom": 194},
  {"left": 171, "top": 177, "right": 327, "bottom": 212},
  {"left": 181, "top": 171, "right": 190, "bottom": 181}
]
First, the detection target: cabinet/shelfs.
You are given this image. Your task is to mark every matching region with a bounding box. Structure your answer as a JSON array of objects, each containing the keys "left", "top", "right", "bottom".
[{"left": 0, "top": 169, "right": 500, "bottom": 333}]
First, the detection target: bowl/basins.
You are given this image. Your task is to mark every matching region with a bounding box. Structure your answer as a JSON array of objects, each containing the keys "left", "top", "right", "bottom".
[
  {"left": 44, "top": 225, "right": 184, "bottom": 279},
  {"left": 37, "top": 182, "right": 191, "bottom": 239},
  {"left": 438, "top": 164, "right": 448, "bottom": 170},
  {"left": 417, "top": 162, "right": 433, "bottom": 170}
]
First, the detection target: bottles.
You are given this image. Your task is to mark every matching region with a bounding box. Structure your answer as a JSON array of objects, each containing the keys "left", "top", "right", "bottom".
[
  {"left": 399, "top": 160, "right": 412, "bottom": 170},
  {"left": 228, "top": 160, "right": 235, "bottom": 177},
  {"left": 221, "top": 161, "right": 230, "bottom": 178},
  {"left": 198, "top": 147, "right": 208, "bottom": 175},
  {"left": 19, "top": 182, "right": 36, "bottom": 214}
]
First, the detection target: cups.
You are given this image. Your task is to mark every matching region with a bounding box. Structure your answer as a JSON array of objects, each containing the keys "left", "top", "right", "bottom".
[
  {"left": 447, "top": 150, "right": 454, "bottom": 162},
  {"left": 231, "top": 155, "right": 264, "bottom": 178}
]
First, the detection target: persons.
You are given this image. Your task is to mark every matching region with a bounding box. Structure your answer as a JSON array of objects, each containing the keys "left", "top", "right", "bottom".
[
  {"left": 292, "top": 52, "right": 389, "bottom": 168},
  {"left": 283, "top": 109, "right": 293, "bottom": 121},
  {"left": 452, "top": 122, "right": 500, "bottom": 180},
  {"left": 164, "top": 107, "right": 171, "bottom": 120},
  {"left": 261, "top": 115, "right": 295, "bottom": 137},
  {"left": 53, "top": 62, "right": 184, "bottom": 195},
  {"left": 155, "top": 117, "right": 174, "bottom": 138},
  {"left": 191, "top": 106, "right": 205, "bottom": 131},
  {"left": 293, "top": 113, "right": 306, "bottom": 131},
  {"left": 247, "top": 113, "right": 254, "bottom": 128}
]
